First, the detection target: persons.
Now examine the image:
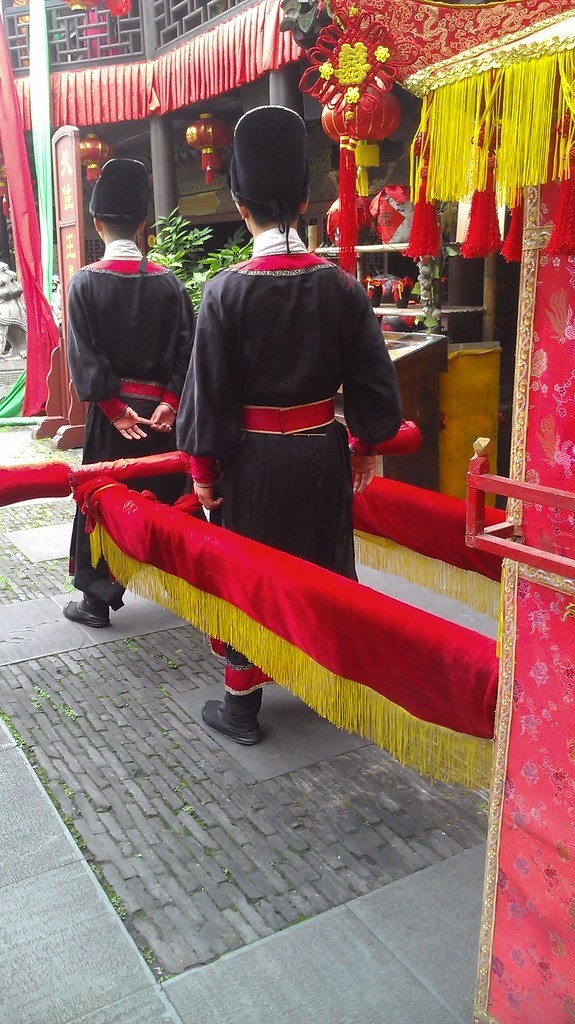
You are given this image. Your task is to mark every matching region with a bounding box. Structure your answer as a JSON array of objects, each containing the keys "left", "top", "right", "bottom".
[
  {"left": 175, "top": 106, "right": 403, "bottom": 746},
  {"left": 63, "top": 158, "right": 197, "bottom": 628}
]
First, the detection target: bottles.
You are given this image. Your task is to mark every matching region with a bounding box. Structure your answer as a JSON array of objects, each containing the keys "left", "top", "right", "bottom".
[
  {"left": 376, "top": 185, "right": 414, "bottom": 245},
  {"left": 362, "top": 278, "right": 416, "bottom": 332},
  {"left": 334, "top": 192, "right": 370, "bottom": 246}
]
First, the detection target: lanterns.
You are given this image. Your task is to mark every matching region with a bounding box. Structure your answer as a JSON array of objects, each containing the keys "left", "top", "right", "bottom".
[
  {"left": 106, "top": 0, "right": 132, "bottom": 16},
  {"left": 185, "top": 112, "right": 231, "bottom": 184},
  {"left": 80, "top": 133, "right": 116, "bottom": 180},
  {"left": 321, "top": 86, "right": 402, "bottom": 196},
  {"left": 62, "top": 0, "right": 103, "bottom": 12}
]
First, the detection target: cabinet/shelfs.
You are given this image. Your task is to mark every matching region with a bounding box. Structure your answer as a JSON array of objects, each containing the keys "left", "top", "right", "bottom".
[{"left": 306, "top": 225, "right": 497, "bottom": 343}]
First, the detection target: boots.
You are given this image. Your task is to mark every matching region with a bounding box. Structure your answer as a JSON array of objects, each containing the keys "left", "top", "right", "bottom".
[
  {"left": 201, "top": 688, "right": 263, "bottom": 745},
  {"left": 64, "top": 593, "right": 110, "bottom": 627}
]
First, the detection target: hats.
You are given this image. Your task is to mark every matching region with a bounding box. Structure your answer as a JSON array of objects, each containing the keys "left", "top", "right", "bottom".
[
  {"left": 89, "top": 159, "right": 149, "bottom": 221},
  {"left": 229, "top": 105, "right": 309, "bottom": 204}
]
